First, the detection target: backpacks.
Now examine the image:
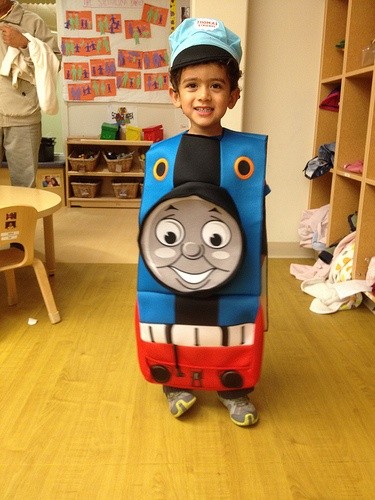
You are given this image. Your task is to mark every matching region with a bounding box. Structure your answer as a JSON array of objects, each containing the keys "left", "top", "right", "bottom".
[{"left": 303, "top": 140, "right": 335, "bottom": 179}]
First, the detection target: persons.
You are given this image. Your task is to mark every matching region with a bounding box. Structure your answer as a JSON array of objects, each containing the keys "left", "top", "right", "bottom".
[
  {"left": 135, "top": 19, "right": 272, "bottom": 428},
  {"left": 1, "top": 0, "right": 63, "bottom": 253}
]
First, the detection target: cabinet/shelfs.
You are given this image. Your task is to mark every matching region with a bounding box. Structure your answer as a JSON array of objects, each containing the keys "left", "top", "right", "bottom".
[
  {"left": 308, "top": 0, "right": 375, "bottom": 315},
  {"left": 66, "top": 137, "right": 154, "bottom": 208}
]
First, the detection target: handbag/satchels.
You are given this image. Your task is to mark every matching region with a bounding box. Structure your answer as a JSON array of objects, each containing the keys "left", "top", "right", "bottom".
[
  {"left": 38, "top": 137, "right": 56, "bottom": 162},
  {"left": 343, "top": 160, "right": 364, "bottom": 175}
]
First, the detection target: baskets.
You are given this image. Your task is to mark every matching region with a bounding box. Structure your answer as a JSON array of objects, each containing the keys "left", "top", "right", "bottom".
[
  {"left": 101, "top": 150, "right": 133, "bottom": 172},
  {"left": 70, "top": 179, "right": 103, "bottom": 198},
  {"left": 111, "top": 180, "right": 139, "bottom": 199},
  {"left": 138, "top": 155, "right": 145, "bottom": 173},
  {"left": 140, "top": 181, "right": 144, "bottom": 194},
  {"left": 68, "top": 148, "right": 100, "bottom": 171}
]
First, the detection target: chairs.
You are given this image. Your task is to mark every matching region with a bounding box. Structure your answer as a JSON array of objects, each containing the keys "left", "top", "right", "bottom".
[{"left": 0, "top": 205, "right": 61, "bottom": 324}]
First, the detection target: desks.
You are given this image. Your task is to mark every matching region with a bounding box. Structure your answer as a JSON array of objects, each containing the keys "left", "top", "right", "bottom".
[{"left": 0, "top": 185, "right": 62, "bottom": 276}]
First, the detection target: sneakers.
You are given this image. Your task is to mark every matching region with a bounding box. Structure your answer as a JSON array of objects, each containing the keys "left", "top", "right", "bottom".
[
  {"left": 216, "top": 392, "right": 258, "bottom": 426},
  {"left": 165, "top": 387, "right": 197, "bottom": 418}
]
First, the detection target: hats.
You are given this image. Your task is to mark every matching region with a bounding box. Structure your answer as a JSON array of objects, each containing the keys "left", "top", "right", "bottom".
[{"left": 168, "top": 18, "right": 242, "bottom": 90}]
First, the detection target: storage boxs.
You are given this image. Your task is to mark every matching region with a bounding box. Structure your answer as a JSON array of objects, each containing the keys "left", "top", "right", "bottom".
[
  {"left": 0, "top": 153, "right": 65, "bottom": 206},
  {"left": 126, "top": 125, "right": 142, "bottom": 141},
  {"left": 142, "top": 124, "right": 163, "bottom": 143},
  {"left": 101, "top": 122, "right": 119, "bottom": 139}
]
